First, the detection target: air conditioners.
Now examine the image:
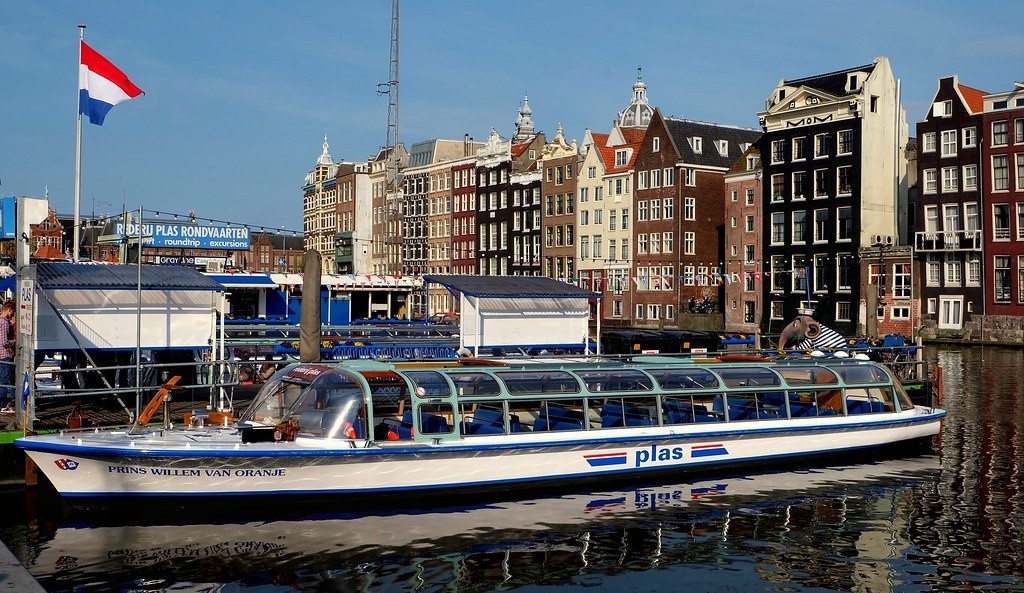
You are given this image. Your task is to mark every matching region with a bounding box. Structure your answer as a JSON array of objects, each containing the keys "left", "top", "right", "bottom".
[
  {"left": 206, "top": 260, "right": 220, "bottom": 272},
  {"left": 884, "top": 236, "right": 894, "bottom": 245},
  {"left": 872, "top": 235, "right": 883, "bottom": 244}
]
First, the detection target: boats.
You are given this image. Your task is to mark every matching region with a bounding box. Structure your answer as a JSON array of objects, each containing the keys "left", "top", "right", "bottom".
[
  {"left": 26, "top": 451, "right": 950, "bottom": 581},
  {"left": 10, "top": 351, "right": 947, "bottom": 504}
]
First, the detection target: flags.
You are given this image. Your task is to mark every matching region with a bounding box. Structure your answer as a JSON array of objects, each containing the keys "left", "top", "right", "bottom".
[
  {"left": 21, "top": 372, "right": 31, "bottom": 411},
  {"left": 79, "top": 39, "right": 145, "bottom": 126}
]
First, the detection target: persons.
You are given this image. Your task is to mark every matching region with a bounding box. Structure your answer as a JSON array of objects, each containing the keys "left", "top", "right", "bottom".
[
  {"left": 259, "top": 353, "right": 290, "bottom": 385},
  {"left": 0, "top": 300, "right": 17, "bottom": 412},
  {"left": 234, "top": 351, "right": 254, "bottom": 385}
]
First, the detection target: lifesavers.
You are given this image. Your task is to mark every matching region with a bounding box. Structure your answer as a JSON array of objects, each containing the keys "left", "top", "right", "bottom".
[{"left": 344, "top": 422, "right": 357, "bottom": 439}]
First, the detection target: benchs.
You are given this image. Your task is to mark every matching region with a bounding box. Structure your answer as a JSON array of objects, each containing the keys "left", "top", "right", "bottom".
[{"left": 375, "top": 390, "right": 887, "bottom": 442}]
